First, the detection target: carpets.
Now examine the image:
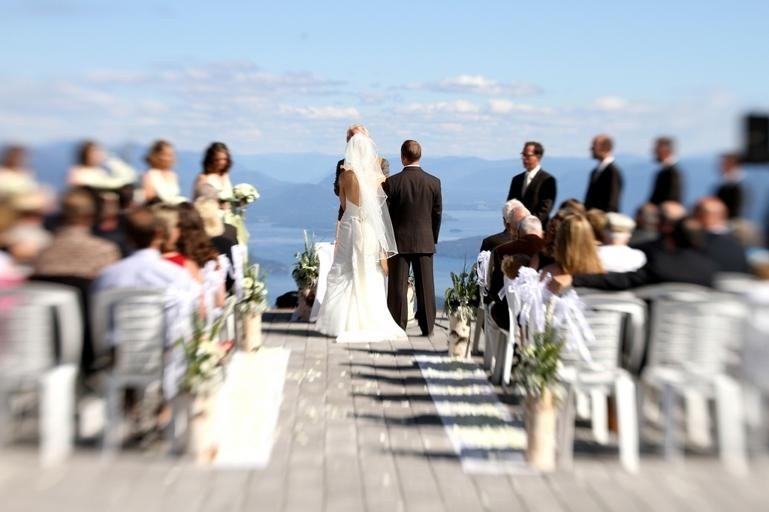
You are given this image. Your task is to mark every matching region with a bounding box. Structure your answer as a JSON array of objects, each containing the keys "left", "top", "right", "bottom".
[
  {"left": 177, "top": 346, "right": 292, "bottom": 469},
  {"left": 410, "top": 352, "right": 533, "bottom": 475}
]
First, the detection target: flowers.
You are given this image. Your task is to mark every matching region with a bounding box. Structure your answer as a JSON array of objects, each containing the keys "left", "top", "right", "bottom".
[
  {"left": 292, "top": 226, "right": 317, "bottom": 289},
  {"left": 442, "top": 252, "right": 482, "bottom": 322},
  {"left": 235, "top": 267, "right": 266, "bottom": 318},
  {"left": 234, "top": 182, "right": 259, "bottom": 202}
]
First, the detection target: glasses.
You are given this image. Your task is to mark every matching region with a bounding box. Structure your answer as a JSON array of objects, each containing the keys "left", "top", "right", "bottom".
[{"left": 520, "top": 152, "right": 538, "bottom": 158}]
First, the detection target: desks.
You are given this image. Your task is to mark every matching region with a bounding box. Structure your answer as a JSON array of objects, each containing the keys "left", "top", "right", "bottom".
[{"left": 314, "top": 242, "right": 417, "bottom": 322}]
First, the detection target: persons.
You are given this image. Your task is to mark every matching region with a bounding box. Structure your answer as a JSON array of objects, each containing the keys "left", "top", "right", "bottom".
[
  {"left": 333, "top": 123, "right": 390, "bottom": 222},
  {"left": 312, "top": 133, "right": 410, "bottom": 342},
  {"left": 647, "top": 134, "right": 686, "bottom": 203},
  {"left": 1, "top": 143, "right": 40, "bottom": 194},
  {"left": 0, "top": 185, "right": 244, "bottom": 452},
  {"left": 68, "top": 138, "right": 138, "bottom": 191},
  {"left": 193, "top": 140, "right": 251, "bottom": 244},
  {"left": 138, "top": 138, "right": 189, "bottom": 206},
  {"left": 504, "top": 140, "right": 559, "bottom": 225},
  {"left": 480, "top": 196, "right": 748, "bottom": 379},
  {"left": 709, "top": 152, "right": 746, "bottom": 219},
  {"left": 381, "top": 140, "right": 443, "bottom": 337},
  {"left": 581, "top": 132, "right": 626, "bottom": 211}
]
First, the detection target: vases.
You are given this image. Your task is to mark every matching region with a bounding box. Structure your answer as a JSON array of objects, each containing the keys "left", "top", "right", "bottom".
[
  {"left": 448, "top": 307, "right": 473, "bottom": 359},
  {"left": 235, "top": 313, "right": 262, "bottom": 352},
  {"left": 297, "top": 290, "right": 311, "bottom": 318}
]
